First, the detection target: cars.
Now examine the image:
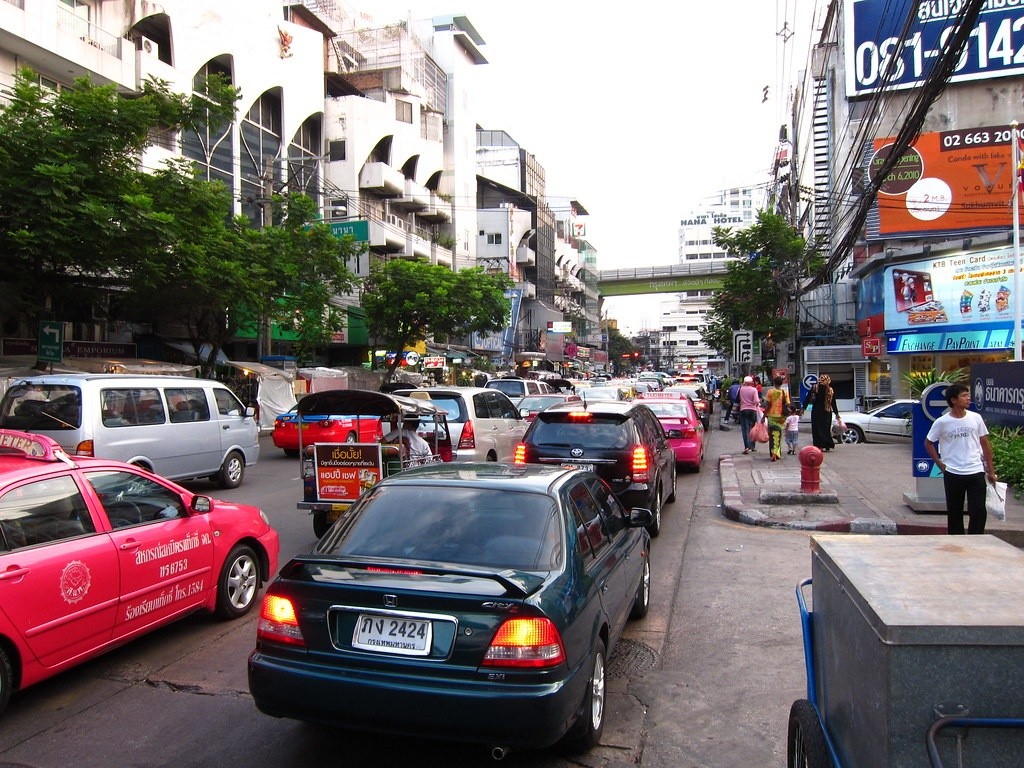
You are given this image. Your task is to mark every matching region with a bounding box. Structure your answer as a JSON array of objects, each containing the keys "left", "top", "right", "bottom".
[
  {"left": 831, "top": 398, "right": 921, "bottom": 444},
  {"left": 483, "top": 379, "right": 556, "bottom": 407},
  {"left": 371, "top": 386, "right": 531, "bottom": 462},
  {"left": 0, "top": 429, "right": 280, "bottom": 713},
  {"left": 512, "top": 400, "right": 683, "bottom": 537},
  {"left": 631, "top": 391, "right": 707, "bottom": 474},
  {"left": 662, "top": 387, "right": 712, "bottom": 431},
  {"left": 270, "top": 403, "right": 383, "bottom": 459},
  {"left": 248, "top": 464, "right": 655, "bottom": 762},
  {"left": 560, "top": 372, "right": 714, "bottom": 413},
  {"left": 515, "top": 395, "right": 582, "bottom": 423}
]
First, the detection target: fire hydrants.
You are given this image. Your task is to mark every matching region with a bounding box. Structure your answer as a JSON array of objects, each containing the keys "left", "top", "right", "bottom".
[{"left": 798, "top": 446, "right": 823, "bottom": 493}]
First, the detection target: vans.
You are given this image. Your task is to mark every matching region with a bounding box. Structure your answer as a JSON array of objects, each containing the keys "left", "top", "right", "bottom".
[{"left": 0, "top": 373, "right": 260, "bottom": 496}]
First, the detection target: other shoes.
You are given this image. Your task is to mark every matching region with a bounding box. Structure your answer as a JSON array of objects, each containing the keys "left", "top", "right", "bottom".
[
  {"left": 787, "top": 450, "right": 792, "bottom": 454},
  {"left": 723, "top": 419, "right": 728, "bottom": 423},
  {"left": 743, "top": 448, "right": 748, "bottom": 454},
  {"left": 771, "top": 454, "right": 776, "bottom": 462},
  {"left": 753, "top": 448, "right": 757, "bottom": 451},
  {"left": 792, "top": 450, "right": 795, "bottom": 454}
]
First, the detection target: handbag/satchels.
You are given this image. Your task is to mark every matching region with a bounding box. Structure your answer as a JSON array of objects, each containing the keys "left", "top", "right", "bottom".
[
  {"left": 749, "top": 421, "right": 769, "bottom": 443},
  {"left": 780, "top": 389, "right": 790, "bottom": 417},
  {"left": 986, "top": 476, "right": 1008, "bottom": 521},
  {"left": 756, "top": 405, "right": 768, "bottom": 426},
  {"left": 832, "top": 418, "right": 848, "bottom": 436}
]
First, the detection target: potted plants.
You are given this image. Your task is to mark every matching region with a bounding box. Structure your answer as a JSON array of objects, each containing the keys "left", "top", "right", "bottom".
[{"left": 719, "top": 378, "right": 733, "bottom": 408}]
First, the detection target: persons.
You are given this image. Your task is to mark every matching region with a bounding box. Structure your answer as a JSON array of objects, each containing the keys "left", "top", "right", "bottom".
[
  {"left": 379, "top": 413, "right": 432, "bottom": 471},
  {"left": 759, "top": 376, "right": 791, "bottom": 459},
  {"left": 798, "top": 374, "right": 841, "bottom": 451},
  {"left": 922, "top": 383, "right": 999, "bottom": 535},
  {"left": 710, "top": 373, "right": 763, "bottom": 424},
  {"left": 783, "top": 405, "right": 804, "bottom": 455},
  {"left": 736, "top": 375, "right": 760, "bottom": 453}
]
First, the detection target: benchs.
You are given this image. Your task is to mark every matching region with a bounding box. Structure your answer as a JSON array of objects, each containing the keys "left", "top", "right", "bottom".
[
  {"left": 104, "top": 398, "right": 211, "bottom": 425},
  {"left": 381, "top": 444, "right": 408, "bottom": 480}
]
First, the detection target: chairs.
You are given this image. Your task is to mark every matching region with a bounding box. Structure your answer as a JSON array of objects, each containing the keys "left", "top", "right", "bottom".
[{"left": 20, "top": 493, "right": 84, "bottom": 546}]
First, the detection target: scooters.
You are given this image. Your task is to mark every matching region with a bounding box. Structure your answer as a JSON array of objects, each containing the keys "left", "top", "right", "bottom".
[{"left": 296, "top": 389, "right": 453, "bottom": 540}]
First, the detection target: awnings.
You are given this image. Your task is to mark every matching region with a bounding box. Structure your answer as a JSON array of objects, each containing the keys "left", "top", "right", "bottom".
[{"left": 1, "top": 355, "right": 421, "bottom": 430}]
